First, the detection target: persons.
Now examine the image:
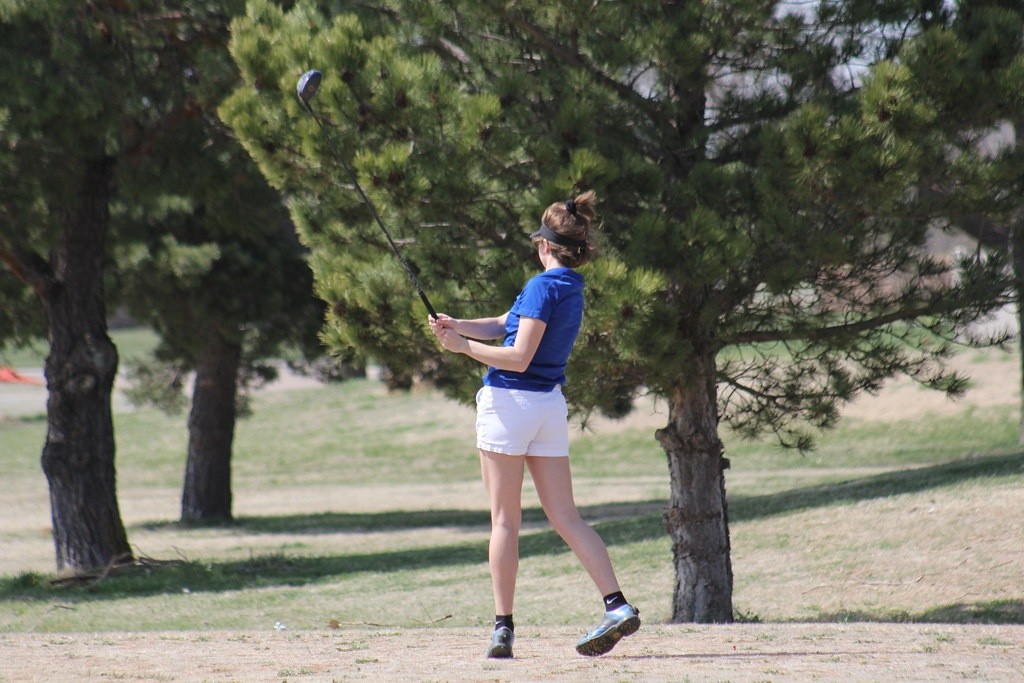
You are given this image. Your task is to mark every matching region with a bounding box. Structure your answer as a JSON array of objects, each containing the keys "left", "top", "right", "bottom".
[{"left": 431, "top": 189, "right": 640, "bottom": 657}]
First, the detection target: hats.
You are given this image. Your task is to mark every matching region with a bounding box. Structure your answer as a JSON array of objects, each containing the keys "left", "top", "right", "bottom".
[{"left": 529, "top": 222, "right": 586, "bottom": 247}]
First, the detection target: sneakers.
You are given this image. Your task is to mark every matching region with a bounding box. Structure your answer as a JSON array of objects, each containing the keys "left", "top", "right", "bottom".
[
  {"left": 486, "top": 626, "right": 515, "bottom": 658},
  {"left": 576, "top": 604, "right": 641, "bottom": 657}
]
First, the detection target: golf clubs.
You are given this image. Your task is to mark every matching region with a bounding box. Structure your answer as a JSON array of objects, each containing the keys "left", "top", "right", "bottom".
[{"left": 298, "top": 69, "right": 447, "bottom": 333}]
